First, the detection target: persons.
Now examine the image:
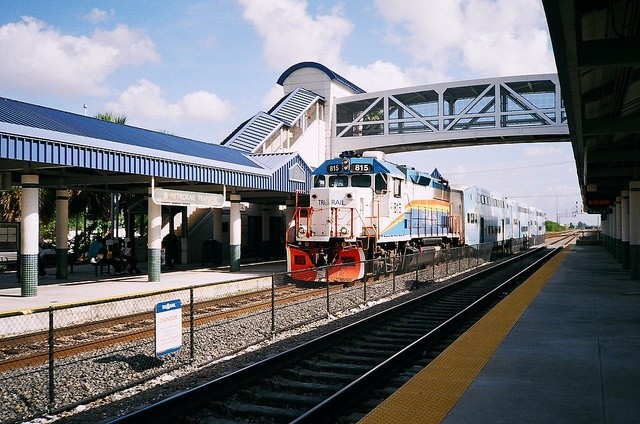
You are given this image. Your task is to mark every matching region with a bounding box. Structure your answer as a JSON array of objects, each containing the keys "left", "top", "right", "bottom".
[
  {"left": 121, "top": 241, "right": 141, "bottom": 274},
  {"left": 88, "top": 236, "right": 111, "bottom": 275},
  {"left": 112, "top": 242, "right": 128, "bottom": 276}
]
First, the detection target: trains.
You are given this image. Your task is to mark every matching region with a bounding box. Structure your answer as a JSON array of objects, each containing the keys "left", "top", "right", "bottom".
[{"left": 286, "top": 150, "right": 546, "bottom": 288}]
